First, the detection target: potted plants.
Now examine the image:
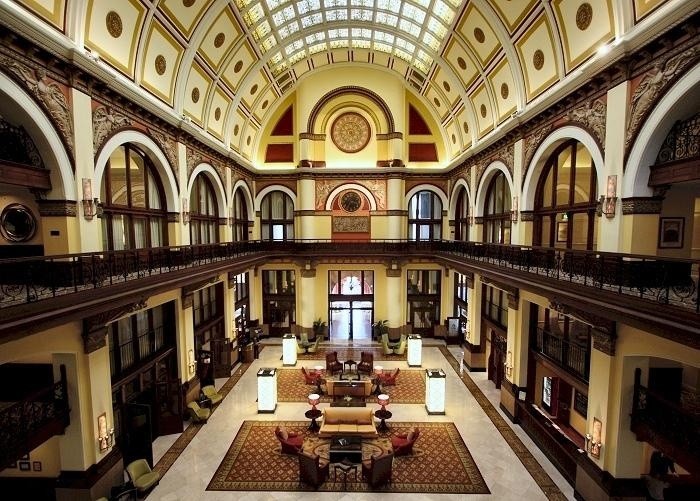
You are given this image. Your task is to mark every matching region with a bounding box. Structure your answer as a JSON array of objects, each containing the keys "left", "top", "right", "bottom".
[
  {"left": 313, "top": 318, "right": 326, "bottom": 342},
  {"left": 371, "top": 320, "right": 389, "bottom": 343}
]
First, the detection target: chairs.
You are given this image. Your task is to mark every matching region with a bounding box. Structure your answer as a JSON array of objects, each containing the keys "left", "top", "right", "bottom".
[
  {"left": 186, "top": 385, "right": 222, "bottom": 424},
  {"left": 97, "top": 458, "right": 161, "bottom": 501},
  {"left": 381, "top": 333, "right": 407, "bottom": 357},
  {"left": 296, "top": 333, "right": 320, "bottom": 355},
  {"left": 277, "top": 345, "right": 421, "bottom": 494}
]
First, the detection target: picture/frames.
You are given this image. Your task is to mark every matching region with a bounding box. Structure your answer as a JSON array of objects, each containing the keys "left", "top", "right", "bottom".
[
  {"left": 8, "top": 453, "right": 41, "bottom": 472},
  {"left": 658, "top": 216, "right": 685, "bottom": 249},
  {"left": 0, "top": 203, "right": 37, "bottom": 242}
]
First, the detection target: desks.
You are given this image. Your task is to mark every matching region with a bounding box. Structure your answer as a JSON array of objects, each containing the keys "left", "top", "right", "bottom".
[
  {"left": 407, "top": 333, "right": 422, "bottom": 367},
  {"left": 283, "top": 334, "right": 298, "bottom": 367}
]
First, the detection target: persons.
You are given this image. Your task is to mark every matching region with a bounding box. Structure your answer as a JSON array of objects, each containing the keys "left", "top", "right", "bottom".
[{"left": 648, "top": 446, "right": 680, "bottom": 481}]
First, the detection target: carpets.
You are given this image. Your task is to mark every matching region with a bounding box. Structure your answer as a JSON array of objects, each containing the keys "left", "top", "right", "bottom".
[
  {"left": 263, "top": 368, "right": 436, "bottom": 404},
  {"left": 298, "top": 343, "right": 395, "bottom": 360},
  {"left": 204, "top": 421, "right": 492, "bottom": 494}
]
